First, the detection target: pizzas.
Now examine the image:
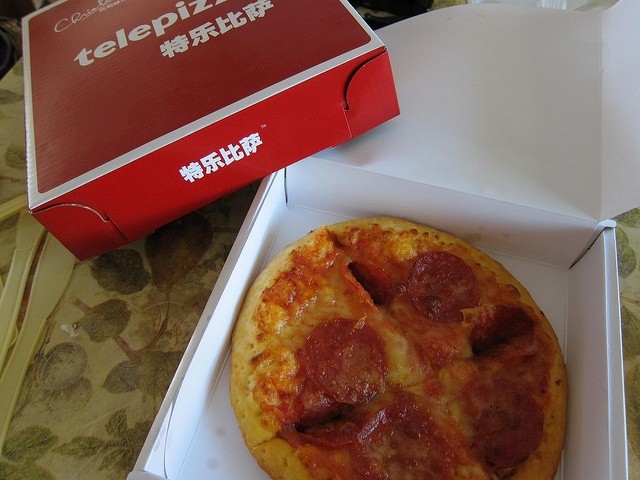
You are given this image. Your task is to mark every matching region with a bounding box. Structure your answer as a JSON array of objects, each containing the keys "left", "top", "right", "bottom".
[{"left": 229, "top": 214, "right": 569, "bottom": 480}]
